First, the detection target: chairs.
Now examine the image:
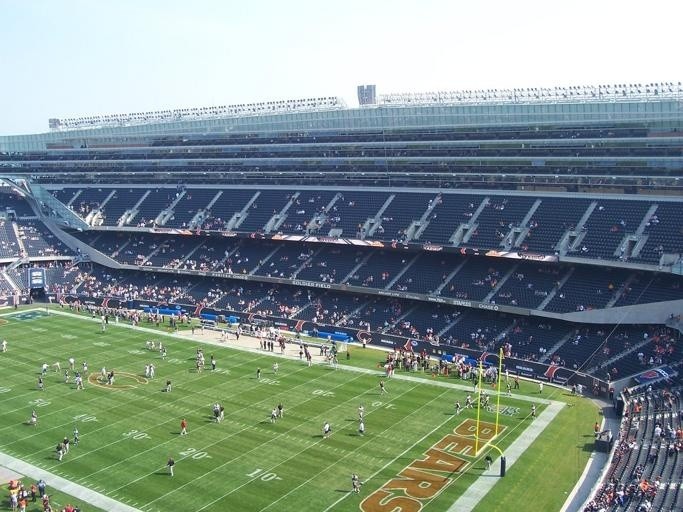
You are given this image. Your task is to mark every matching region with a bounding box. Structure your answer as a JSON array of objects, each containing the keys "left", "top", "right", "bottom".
[
  {"left": 1, "top": 128, "right": 683, "bottom": 512},
  {"left": 574, "top": 384, "right": 681, "bottom": 512}
]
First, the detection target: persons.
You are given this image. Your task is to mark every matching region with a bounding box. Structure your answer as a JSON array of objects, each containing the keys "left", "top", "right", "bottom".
[{"left": 0, "top": 186, "right": 683, "bottom": 512}]
